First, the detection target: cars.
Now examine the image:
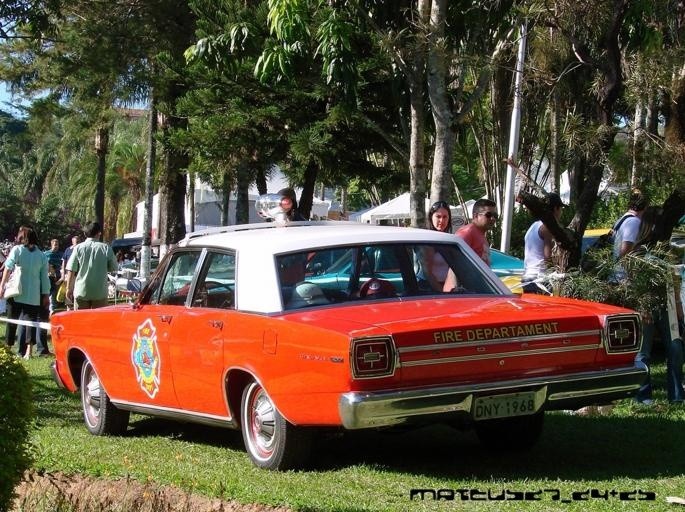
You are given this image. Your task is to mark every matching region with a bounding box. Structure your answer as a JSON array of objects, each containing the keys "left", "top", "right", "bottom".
[
  {"left": 110, "top": 236, "right": 160, "bottom": 271},
  {"left": 166, "top": 239, "right": 524, "bottom": 306},
  {"left": 49, "top": 193, "right": 650, "bottom": 472},
  {"left": 578, "top": 229, "right": 612, "bottom": 272}
]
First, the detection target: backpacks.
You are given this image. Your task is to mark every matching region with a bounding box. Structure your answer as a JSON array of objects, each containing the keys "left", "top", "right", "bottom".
[{"left": 582, "top": 215, "right": 634, "bottom": 280}]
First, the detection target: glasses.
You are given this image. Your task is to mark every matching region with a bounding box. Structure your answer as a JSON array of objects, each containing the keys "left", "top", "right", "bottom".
[{"left": 479, "top": 212, "right": 498, "bottom": 218}]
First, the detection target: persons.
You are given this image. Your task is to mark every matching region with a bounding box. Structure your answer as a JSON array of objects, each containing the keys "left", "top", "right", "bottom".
[
  {"left": 0, "top": 224, "right": 51, "bottom": 362},
  {"left": 416, "top": 201, "right": 453, "bottom": 293},
  {"left": 0, "top": 238, "right": 141, "bottom": 311},
  {"left": 16, "top": 306, "right": 54, "bottom": 359},
  {"left": 65, "top": 221, "right": 118, "bottom": 310},
  {"left": 265, "top": 186, "right": 309, "bottom": 221},
  {"left": 604, "top": 187, "right": 658, "bottom": 406},
  {"left": 521, "top": 193, "right": 569, "bottom": 294},
  {"left": 443, "top": 199, "right": 497, "bottom": 292}
]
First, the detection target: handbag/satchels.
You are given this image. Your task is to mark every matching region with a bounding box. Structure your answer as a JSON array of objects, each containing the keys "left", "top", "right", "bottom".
[
  {"left": 3, "top": 266, "right": 22, "bottom": 299},
  {"left": 56, "top": 281, "right": 65, "bottom": 303}
]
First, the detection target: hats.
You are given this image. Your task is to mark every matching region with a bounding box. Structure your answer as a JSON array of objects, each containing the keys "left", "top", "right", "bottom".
[{"left": 545, "top": 193, "right": 569, "bottom": 207}]
